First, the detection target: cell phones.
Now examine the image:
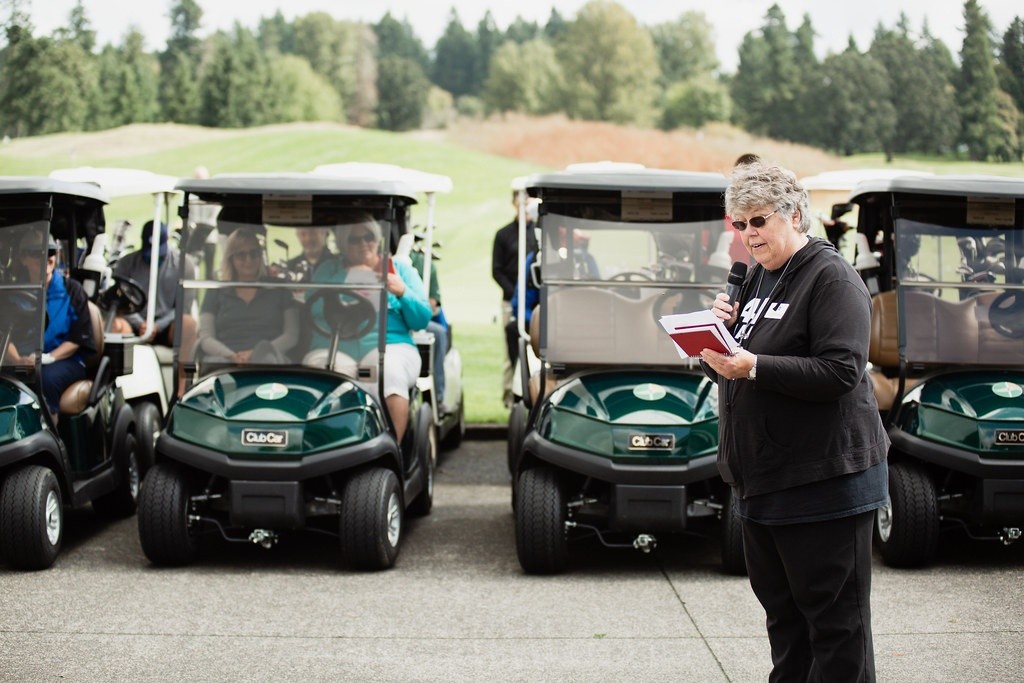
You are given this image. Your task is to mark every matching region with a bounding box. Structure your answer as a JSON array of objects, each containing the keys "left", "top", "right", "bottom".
[{"left": 379, "top": 252, "right": 395, "bottom": 275}]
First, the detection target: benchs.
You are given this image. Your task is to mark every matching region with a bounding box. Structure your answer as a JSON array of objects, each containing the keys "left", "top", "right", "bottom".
[
  {"left": 152, "top": 300, "right": 200, "bottom": 365},
  {"left": 59, "top": 300, "right": 105, "bottom": 414},
  {"left": 867, "top": 291, "right": 1024, "bottom": 410},
  {"left": 529, "top": 288, "right": 718, "bottom": 407}
]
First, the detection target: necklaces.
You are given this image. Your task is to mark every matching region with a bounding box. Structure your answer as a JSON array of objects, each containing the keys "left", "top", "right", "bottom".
[{"left": 737, "top": 238, "right": 807, "bottom": 348}]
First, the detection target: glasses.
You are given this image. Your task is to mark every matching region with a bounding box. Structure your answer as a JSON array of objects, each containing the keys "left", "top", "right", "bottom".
[
  {"left": 349, "top": 234, "right": 372, "bottom": 244},
  {"left": 732, "top": 205, "right": 789, "bottom": 231},
  {"left": 25, "top": 249, "right": 56, "bottom": 258},
  {"left": 233, "top": 249, "right": 260, "bottom": 260}
]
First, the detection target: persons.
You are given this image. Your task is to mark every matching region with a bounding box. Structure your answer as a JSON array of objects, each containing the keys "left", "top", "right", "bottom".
[
  {"left": 492, "top": 187, "right": 539, "bottom": 409},
  {"left": 810, "top": 210, "right": 874, "bottom": 267},
  {"left": 285, "top": 225, "right": 335, "bottom": 284},
  {"left": 510, "top": 218, "right": 600, "bottom": 331},
  {"left": 301, "top": 213, "right": 433, "bottom": 446},
  {"left": 701, "top": 152, "right": 761, "bottom": 278},
  {"left": 197, "top": 227, "right": 301, "bottom": 365},
  {"left": 700, "top": 161, "right": 888, "bottom": 683},
  {"left": 110, "top": 219, "right": 197, "bottom": 399},
  {"left": 0, "top": 230, "right": 96, "bottom": 427},
  {"left": 409, "top": 249, "right": 452, "bottom": 420}
]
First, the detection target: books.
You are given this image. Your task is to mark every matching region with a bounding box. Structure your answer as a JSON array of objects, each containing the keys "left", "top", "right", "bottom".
[{"left": 668, "top": 323, "right": 733, "bottom": 359}]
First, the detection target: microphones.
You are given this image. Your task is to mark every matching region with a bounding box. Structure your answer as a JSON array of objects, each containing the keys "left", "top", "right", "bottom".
[{"left": 723, "top": 262, "right": 747, "bottom": 328}]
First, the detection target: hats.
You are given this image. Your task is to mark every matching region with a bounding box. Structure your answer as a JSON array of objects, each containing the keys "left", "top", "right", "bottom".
[{"left": 19, "top": 229, "right": 62, "bottom": 251}]
